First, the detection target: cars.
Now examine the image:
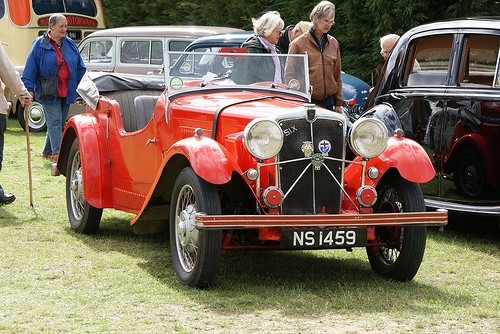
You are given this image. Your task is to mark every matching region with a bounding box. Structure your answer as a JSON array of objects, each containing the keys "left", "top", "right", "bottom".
[
  {"left": 56, "top": 51, "right": 450, "bottom": 284},
  {"left": 72, "top": 25, "right": 372, "bottom": 113},
  {"left": 363, "top": 17, "right": 500, "bottom": 217}
]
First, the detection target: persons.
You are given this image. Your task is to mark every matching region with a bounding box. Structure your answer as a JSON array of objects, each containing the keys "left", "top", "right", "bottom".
[
  {"left": 231, "top": 0, "right": 420, "bottom": 115},
  {"left": 0, "top": 42, "right": 32, "bottom": 203},
  {"left": 21, "top": 13, "right": 86, "bottom": 163}
]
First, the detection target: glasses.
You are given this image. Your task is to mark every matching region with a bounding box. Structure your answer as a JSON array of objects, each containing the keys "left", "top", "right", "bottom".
[{"left": 275, "top": 29, "right": 285, "bottom": 36}]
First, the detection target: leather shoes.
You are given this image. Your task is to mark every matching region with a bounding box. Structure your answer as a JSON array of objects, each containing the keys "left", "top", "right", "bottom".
[{"left": 0, "top": 192, "right": 15, "bottom": 204}]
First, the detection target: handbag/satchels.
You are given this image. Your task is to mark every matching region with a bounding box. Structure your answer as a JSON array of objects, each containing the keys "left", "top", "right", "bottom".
[{"left": 39, "top": 75, "right": 58, "bottom": 100}]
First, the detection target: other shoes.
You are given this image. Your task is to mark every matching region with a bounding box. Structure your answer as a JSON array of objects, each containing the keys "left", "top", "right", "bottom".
[
  {"left": 50, "top": 154, "right": 59, "bottom": 162},
  {"left": 42, "top": 154, "right": 52, "bottom": 160}
]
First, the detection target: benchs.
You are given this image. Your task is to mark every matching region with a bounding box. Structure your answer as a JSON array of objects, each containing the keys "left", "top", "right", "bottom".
[
  {"left": 406, "top": 73, "right": 494, "bottom": 88},
  {"left": 100, "top": 90, "right": 163, "bottom": 132}
]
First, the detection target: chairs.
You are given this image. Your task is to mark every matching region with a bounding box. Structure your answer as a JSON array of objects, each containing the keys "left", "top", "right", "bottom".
[{"left": 134, "top": 95, "right": 160, "bottom": 130}]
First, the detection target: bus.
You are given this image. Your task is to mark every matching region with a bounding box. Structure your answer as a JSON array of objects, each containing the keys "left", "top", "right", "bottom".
[{"left": 0, "top": 0, "right": 106, "bottom": 128}]
